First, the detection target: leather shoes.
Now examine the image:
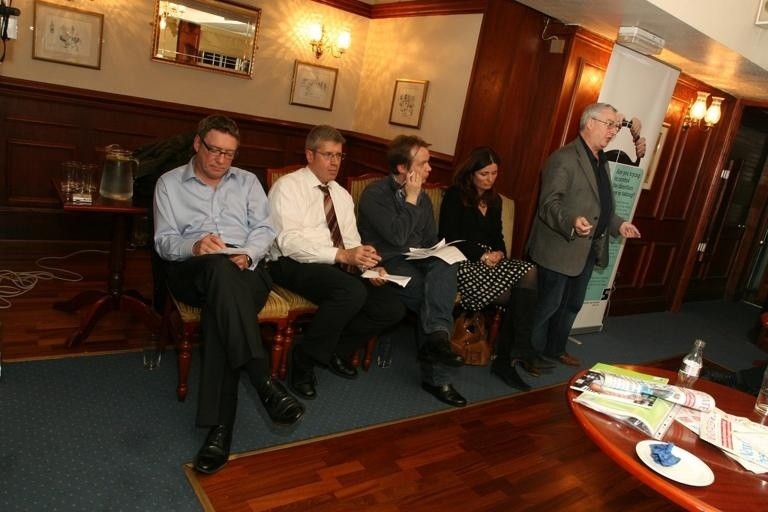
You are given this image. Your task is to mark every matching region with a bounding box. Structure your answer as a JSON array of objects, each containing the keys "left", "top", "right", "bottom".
[
  {"left": 418, "top": 334, "right": 463, "bottom": 368},
  {"left": 311, "top": 352, "right": 357, "bottom": 379},
  {"left": 557, "top": 352, "right": 581, "bottom": 367},
  {"left": 249, "top": 344, "right": 316, "bottom": 432},
  {"left": 194, "top": 425, "right": 233, "bottom": 475},
  {"left": 421, "top": 375, "right": 467, "bottom": 407}
]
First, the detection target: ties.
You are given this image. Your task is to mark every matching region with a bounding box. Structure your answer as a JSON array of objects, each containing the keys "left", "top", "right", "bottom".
[{"left": 319, "top": 184, "right": 354, "bottom": 273}]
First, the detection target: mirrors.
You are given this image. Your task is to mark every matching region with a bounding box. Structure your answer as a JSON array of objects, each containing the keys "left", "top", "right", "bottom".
[{"left": 150, "top": 0, "right": 262, "bottom": 81}]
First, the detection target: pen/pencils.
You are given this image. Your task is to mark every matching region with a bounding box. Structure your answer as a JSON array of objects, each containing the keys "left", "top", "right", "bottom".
[{"left": 399, "top": 171, "right": 415, "bottom": 187}]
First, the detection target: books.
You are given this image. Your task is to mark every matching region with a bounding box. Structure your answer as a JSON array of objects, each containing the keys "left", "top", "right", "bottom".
[{"left": 570, "top": 362, "right": 767, "bottom": 474}]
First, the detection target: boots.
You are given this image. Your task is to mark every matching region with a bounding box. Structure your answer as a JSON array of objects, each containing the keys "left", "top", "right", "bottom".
[{"left": 490, "top": 287, "right": 557, "bottom": 392}]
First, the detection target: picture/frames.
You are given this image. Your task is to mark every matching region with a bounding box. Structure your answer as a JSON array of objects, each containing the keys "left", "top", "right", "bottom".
[
  {"left": 640, "top": 123, "right": 672, "bottom": 196},
  {"left": 386, "top": 78, "right": 429, "bottom": 129},
  {"left": 288, "top": 60, "right": 339, "bottom": 111},
  {"left": 32, "top": 1, "right": 104, "bottom": 70}
]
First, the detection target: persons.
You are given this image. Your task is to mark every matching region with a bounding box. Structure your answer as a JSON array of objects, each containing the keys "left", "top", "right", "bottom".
[
  {"left": 358, "top": 135, "right": 468, "bottom": 408},
  {"left": 634, "top": 137, "right": 646, "bottom": 158},
  {"left": 265, "top": 124, "right": 389, "bottom": 400},
  {"left": 153, "top": 113, "right": 305, "bottom": 476},
  {"left": 523, "top": 102, "right": 641, "bottom": 377},
  {"left": 630, "top": 117, "right": 641, "bottom": 140},
  {"left": 616, "top": 112, "right": 624, "bottom": 130},
  {"left": 438, "top": 146, "right": 556, "bottom": 393}
]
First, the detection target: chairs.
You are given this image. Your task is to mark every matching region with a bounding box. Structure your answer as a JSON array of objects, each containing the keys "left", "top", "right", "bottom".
[
  {"left": 147, "top": 165, "right": 290, "bottom": 402},
  {"left": 485, "top": 193, "right": 516, "bottom": 356},
  {"left": 265, "top": 164, "right": 360, "bottom": 380},
  {"left": 345, "top": 172, "right": 389, "bottom": 370},
  {"left": 420, "top": 181, "right": 447, "bottom": 238}
]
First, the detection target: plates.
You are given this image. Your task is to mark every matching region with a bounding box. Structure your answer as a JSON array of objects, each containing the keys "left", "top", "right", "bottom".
[{"left": 636, "top": 438, "right": 714, "bottom": 487}]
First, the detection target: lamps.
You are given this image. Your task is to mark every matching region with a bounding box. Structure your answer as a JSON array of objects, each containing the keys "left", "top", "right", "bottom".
[
  {"left": 304, "top": 21, "right": 352, "bottom": 60},
  {"left": 616, "top": 27, "right": 665, "bottom": 59},
  {"left": 683, "top": 91, "right": 727, "bottom": 130},
  {"left": 3, "top": 5, "right": 20, "bottom": 44}
]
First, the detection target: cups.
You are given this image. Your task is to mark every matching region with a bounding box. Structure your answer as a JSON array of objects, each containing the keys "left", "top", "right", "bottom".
[
  {"left": 375, "top": 341, "right": 394, "bottom": 367},
  {"left": 142, "top": 336, "right": 162, "bottom": 370},
  {"left": 62, "top": 161, "right": 98, "bottom": 195},
  {"left": 752, "top": 366, "right": 768, "bottom": 416}
]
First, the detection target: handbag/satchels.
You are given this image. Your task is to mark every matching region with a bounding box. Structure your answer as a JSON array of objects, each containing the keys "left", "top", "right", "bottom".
[{"left": 449, "top": 333, "right": 490, "bottom": 366}]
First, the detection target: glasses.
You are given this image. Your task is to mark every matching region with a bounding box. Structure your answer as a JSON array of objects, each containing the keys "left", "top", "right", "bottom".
[
  {"left": 308, "top": 147, "right": 347, "bottom": 163},
  {"left": 198, "top": 137, "right": 239, "bottom": 159},
  {"left": 592, "top": 117, "right": 620, "bottom": 132}
]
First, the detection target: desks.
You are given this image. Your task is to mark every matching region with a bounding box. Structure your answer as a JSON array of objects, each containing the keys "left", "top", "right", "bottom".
[
  {"left": 565, "top": 363, "right": 768, "bottom": 511},
  {"left": 50, "top": 176, "right": 147, "bottom": 350}
]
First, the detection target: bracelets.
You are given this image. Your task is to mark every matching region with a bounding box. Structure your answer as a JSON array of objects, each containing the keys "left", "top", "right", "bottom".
[
  {"left": 483, "top": 251, "right": 489, "bottom": 263},
  {"left": 246, "top": 255, "right": 253, "bottom": 267}
]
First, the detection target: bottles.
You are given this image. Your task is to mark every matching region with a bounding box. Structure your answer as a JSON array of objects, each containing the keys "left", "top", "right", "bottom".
[{"left": 674, "top": 340, "right": 705, "bottom": 389}]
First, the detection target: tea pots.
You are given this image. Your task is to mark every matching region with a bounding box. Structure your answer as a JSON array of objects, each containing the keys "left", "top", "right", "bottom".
[{"left": 98, "top": 144, "right": 140, "bottom": 200}]
